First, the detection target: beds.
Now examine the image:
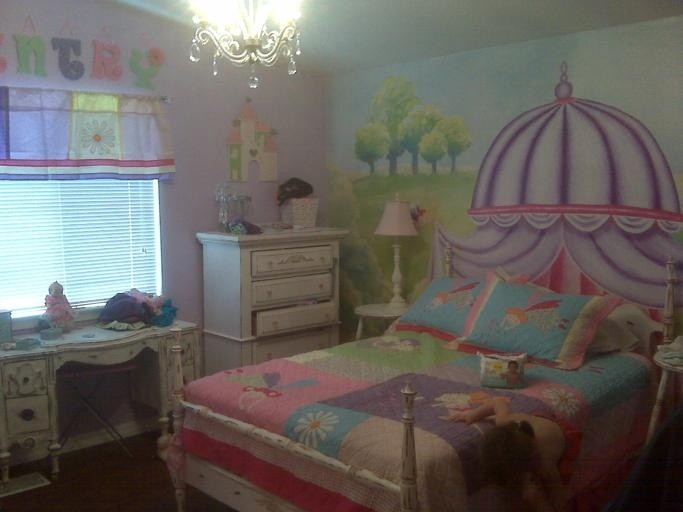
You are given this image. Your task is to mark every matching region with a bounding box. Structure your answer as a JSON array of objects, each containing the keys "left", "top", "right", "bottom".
[{"left": 170, "top": 242, "right": 676, "bottom": 512}]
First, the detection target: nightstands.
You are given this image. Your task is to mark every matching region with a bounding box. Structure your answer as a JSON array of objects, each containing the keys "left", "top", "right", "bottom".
[
  {"left": 645, "top": 342, "right": 683, "bottom": 443},
  {"left": 353, "top": 304, "right": 411, "bottom": 340}
]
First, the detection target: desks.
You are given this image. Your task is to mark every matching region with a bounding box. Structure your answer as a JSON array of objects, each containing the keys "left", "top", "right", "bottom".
[{"left": 0, "top": 318, "right": 199, "bottom": 494}]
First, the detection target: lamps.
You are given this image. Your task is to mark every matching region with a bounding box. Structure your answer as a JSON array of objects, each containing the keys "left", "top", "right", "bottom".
[
  {"left": 373, "top": 194, "right": 418, "bottom": 304},
  {"left": 186, "top": 0, "right": 305, "bottom": 90}
]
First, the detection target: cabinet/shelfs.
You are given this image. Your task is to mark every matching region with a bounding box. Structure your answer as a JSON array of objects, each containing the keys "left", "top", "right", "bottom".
[{"left": 197, "top": 225, "right": 351, "bottom": 374}]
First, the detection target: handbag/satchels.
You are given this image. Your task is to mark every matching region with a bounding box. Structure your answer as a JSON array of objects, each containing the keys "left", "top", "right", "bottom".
[{"left": 276, "top": 177, "right": 314, "bottom": 207}]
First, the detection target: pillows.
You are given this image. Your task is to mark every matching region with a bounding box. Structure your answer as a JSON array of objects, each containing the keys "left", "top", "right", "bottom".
[
  {"left": 391, "top": 277, "right": 489, "bottom": 340},
  {"left": 444, "top": 271, "right": 620, "bottom": 371}
]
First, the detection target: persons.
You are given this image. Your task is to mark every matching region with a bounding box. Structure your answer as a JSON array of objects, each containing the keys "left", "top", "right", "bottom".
[
  {"left": 447, "top": 397, "right": 583, "bottom": 512},
  {"left": 501, "top": 361, "right": 522, "bottom": 385}
]
